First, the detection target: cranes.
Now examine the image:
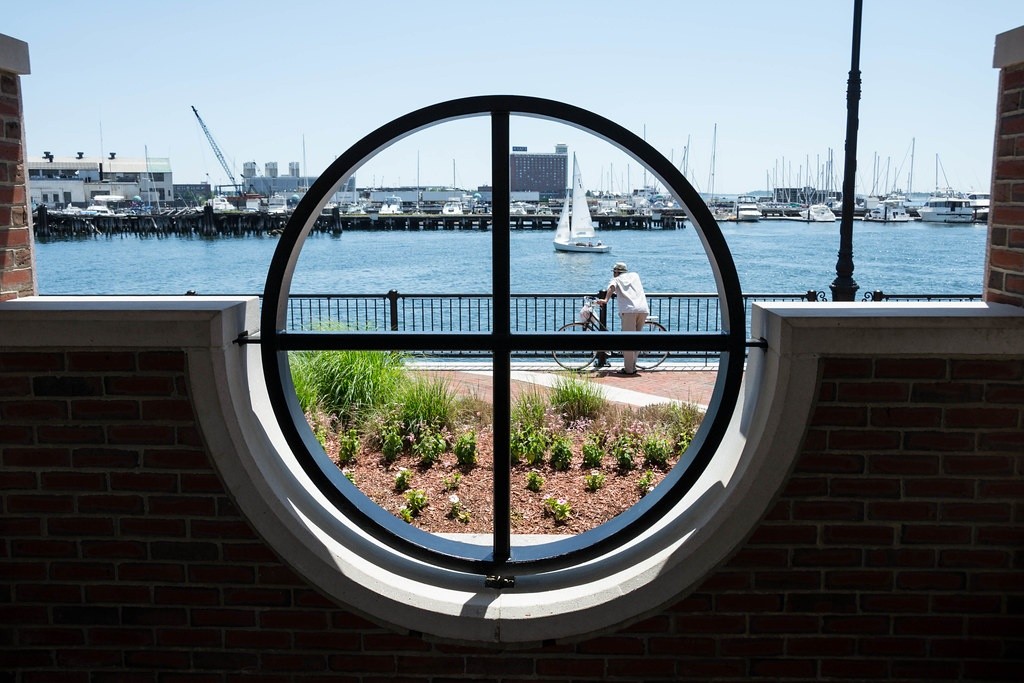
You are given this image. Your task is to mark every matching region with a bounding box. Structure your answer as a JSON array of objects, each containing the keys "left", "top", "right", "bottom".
[{"left": 191, "top": 104, "right": 275, "bottom": 203}]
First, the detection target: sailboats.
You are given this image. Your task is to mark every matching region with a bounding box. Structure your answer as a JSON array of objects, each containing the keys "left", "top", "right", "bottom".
[
  {"left": 33, "top": 123, "right": 990, "bottom": 223},
  {"left": 552, "top": 150, "right": 613, "bottom": 253}
]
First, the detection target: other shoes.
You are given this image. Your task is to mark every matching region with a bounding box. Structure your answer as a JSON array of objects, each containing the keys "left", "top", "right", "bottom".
[{"left": 618, "top": 368, "right": 637, "bottom": 375}]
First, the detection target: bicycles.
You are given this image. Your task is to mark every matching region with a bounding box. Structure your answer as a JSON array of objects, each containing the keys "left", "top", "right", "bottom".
[{"left": 553, "top": 296, "right": 669, "bottom": 370}]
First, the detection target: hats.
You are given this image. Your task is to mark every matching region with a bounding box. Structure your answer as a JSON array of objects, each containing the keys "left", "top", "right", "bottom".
[{"left": 611, "top": 263, "right": 628, "bottom": 272}]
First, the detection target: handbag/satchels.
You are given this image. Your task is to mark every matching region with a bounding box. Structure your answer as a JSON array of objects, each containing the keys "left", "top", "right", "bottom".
[{"left": 580, "top": 303, "right": 599, "bottom": 323}]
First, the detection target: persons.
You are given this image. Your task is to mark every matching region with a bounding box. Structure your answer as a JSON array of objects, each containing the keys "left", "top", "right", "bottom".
[{"left": 596, "top": 263, "right": 650, "bottom": 374}]
